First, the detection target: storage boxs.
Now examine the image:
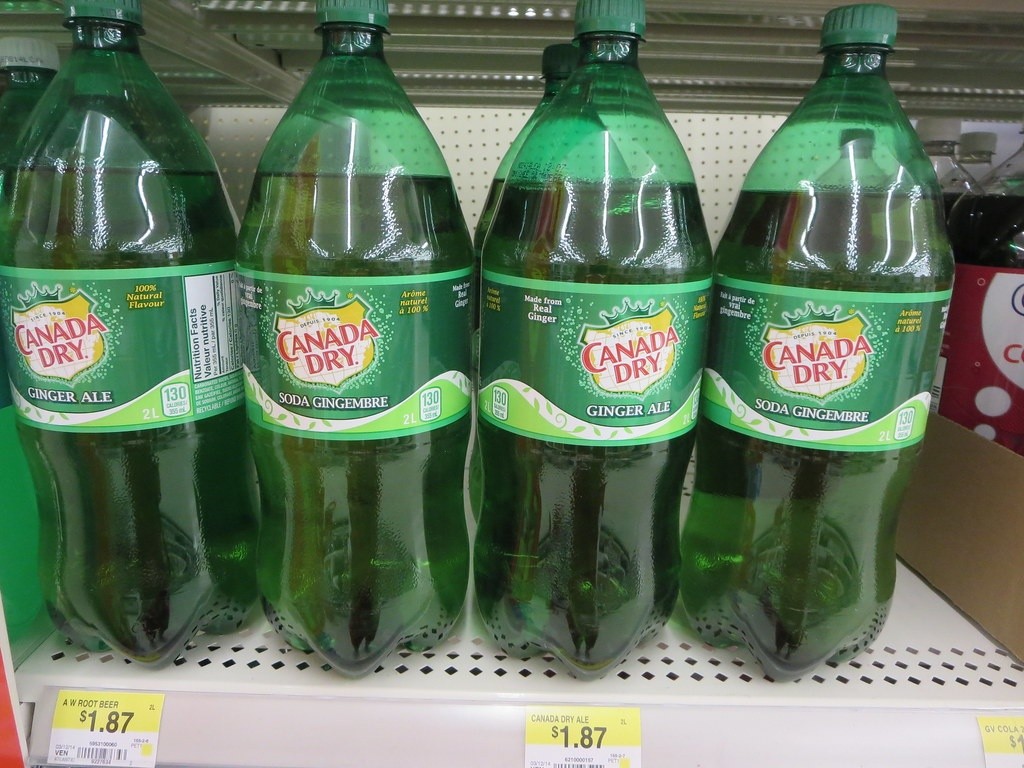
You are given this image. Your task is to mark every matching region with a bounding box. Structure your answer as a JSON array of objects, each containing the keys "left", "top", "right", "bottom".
[
  {"left": 886, "top": 409, "right": 1024, "bottom": 665},
  {"left": 941, "top": 265, "right": 1024, "bottom": 454}
]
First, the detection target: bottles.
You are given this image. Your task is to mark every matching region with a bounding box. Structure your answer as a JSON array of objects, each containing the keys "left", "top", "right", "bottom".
[{"left": 0, "top": 0, "right": 1023, "bottom": 681}]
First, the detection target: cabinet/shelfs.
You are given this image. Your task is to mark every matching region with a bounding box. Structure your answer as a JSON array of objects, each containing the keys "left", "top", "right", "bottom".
[{"left": 0, "top": 0, "right": 1024, "bottom": 768}]
